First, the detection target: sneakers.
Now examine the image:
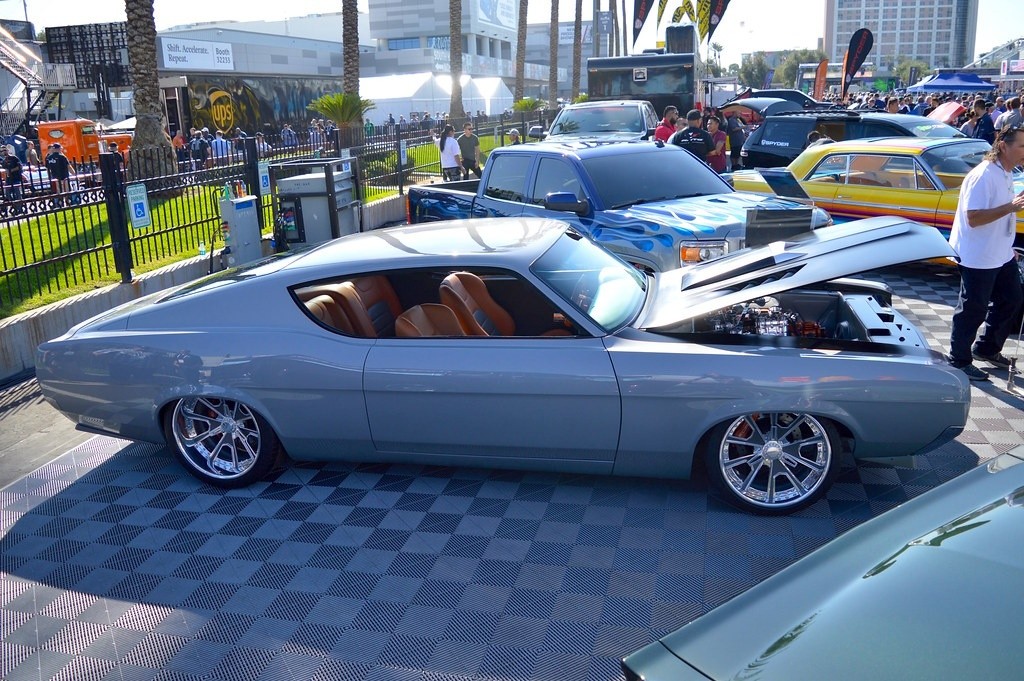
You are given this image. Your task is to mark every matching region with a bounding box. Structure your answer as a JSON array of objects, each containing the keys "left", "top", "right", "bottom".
[
  {"left": 956, "top": 364, "right": 989, "bottom": 380},
  {"left": 971, "top": 344, "right": 1011, "bottom": 367}
]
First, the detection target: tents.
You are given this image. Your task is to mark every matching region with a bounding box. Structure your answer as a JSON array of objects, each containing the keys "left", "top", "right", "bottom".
[
  {"left": 906, "top": 73, "right": 996, "bottom": 98},
  {"left": 359, "top": 72, "right": 514, "bottom": 124}
]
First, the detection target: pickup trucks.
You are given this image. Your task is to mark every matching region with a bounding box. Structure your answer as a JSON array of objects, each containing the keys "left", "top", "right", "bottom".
[{"left": 406, "top": 140, "right": 832, "bottom": 275}]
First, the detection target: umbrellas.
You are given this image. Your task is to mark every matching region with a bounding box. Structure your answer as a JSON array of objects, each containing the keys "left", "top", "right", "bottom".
[{"left": 83, "top": 115, "right": 138, "bottom": 131}]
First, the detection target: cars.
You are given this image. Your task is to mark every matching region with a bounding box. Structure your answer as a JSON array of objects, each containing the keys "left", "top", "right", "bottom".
[
  {"left": 34, "top": 215, "right": 971, "bottom": 515},
  {"left": 718, "top": 136, "right": 1024, "bottom": 289}
]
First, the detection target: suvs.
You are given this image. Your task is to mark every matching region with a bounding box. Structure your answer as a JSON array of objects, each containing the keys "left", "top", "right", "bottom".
[
  {"left": 717, "top": 88, "right": 970, "bottom": 170},
  {"left": 529, "top": 98, "right": 660, "bottom": 143}
]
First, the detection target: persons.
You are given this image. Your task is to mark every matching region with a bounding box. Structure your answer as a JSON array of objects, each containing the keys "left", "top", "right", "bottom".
[
  {"left": 364, "top": 111, "right": 448, "bottom": 149},
  {"left": 466, "top": 108, "right": 513, "bottom": 127},
  {"left": 505, "top": 129, "right": 522, "bottom": 145},
  {"left": 457, "top": 121, "right": 482, "bottom": 180},
  {"left": 0, "top": 141, "right": 123, "bottom": 216},
  {"left": 809, "top": 87, "right": 1024, "bottom": 145},
  {"left": 655, "top": 105, "right": 750, "bottom": 175},
  {"left": 173, "top": 118, "right": 337, "bottom": 181},
  {"left": 429, "top": 124, "right": 466, "bottom": 181},
  {"left": 949, "top": 121, "right": 1024, "bottom": 380}
]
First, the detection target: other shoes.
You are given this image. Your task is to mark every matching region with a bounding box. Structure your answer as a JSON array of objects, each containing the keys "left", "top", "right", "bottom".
[{"left": 731, "top": 164, "right": 744, "bottom": 170}]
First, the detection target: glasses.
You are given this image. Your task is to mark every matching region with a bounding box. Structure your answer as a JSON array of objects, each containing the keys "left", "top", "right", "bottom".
[
  {"left": 1001, "top": 122, "right": 1020, "bottom": 140},
  {"left": 467, "top": 128, "right": 471, "bottom": 131},
  {"left": 1, "top": 149, "right": 6, "bottom": 152},
  {"left": 672, "top": 111, "right": 678, "bottom": 115}
]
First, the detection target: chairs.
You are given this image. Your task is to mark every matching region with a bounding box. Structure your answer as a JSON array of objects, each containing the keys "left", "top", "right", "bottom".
[
  {"left": 293, "top": 269, "right": 576, "bottom": 338},
  {"left": 815, "top": 171, "right": 934, "bottom": 188}
]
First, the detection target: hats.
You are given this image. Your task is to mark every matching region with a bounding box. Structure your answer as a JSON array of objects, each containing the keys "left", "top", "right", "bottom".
[
  {"left": 201, "top": 127, "right": 209, "bottom": 133},
  {"left": 109, "top": 142, "right": 117, "bottom": 147},
  {"left": 54, "top": 143, "right": 60, "bottom": 148},
  {"left": 47, "top": 144, "right": 53, "bottom": 149},
  {"left": 505, "top": 129, "right": 520, "bottom": 135},
  {"left": 255, "top": 131, "right": 264, "bottom": 136},
  {"left": 996, "top": 97, "right": 1005, "bottom": 102},
  {"left": 686, "top": 109, "right": 704, "bottom": 120}
]
